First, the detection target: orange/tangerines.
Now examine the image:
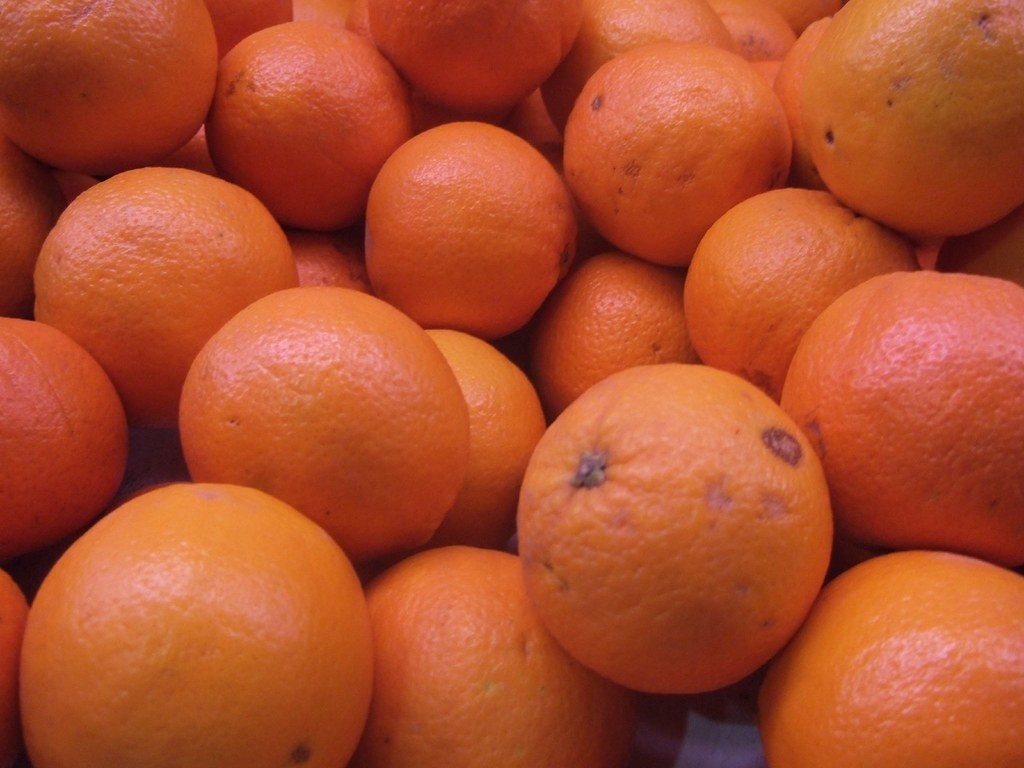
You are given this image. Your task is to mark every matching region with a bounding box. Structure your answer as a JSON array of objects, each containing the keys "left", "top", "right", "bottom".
[{"left": 0, "top": 0, "right": 1024, "bottom": 768}]
